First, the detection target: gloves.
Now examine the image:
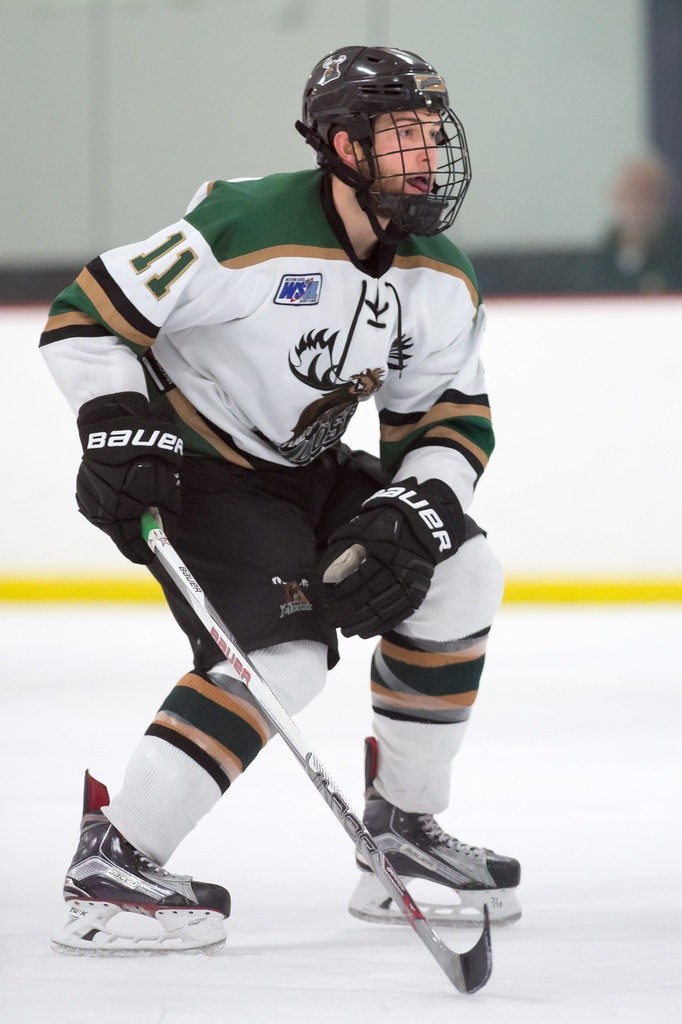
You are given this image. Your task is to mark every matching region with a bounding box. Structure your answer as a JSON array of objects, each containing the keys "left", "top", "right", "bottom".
[
  {"left": 74, "top": 391, "right": 183, "bottom": 567},
  {"left": 308, "top": 475, "right": 467, "bottom": 638}
]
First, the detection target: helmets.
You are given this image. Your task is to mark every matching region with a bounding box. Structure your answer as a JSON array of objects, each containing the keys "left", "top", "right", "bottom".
[{"left": 294, "top": 44, "right": 449, "bottom": 236}]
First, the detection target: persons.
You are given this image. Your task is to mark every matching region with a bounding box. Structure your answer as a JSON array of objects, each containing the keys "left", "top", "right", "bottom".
[
  {"left": 38, "top": 46, "right": 523, "bottom": 957},
  {"left": 593, "top": 157, "right": 682, "bottom": 292}
]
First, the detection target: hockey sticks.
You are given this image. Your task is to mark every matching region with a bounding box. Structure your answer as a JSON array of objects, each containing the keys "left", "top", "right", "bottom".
[{"left": 139, "top": 509, "right": 496, "bottom": 993}]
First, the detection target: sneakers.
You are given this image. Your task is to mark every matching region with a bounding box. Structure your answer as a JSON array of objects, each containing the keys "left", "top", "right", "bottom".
[
  {"left": 48, "top": 768, "right": 231, "bottom": 956},
  {"left": 348, "top": 736, "right": 521, "bottom": 929}
]
